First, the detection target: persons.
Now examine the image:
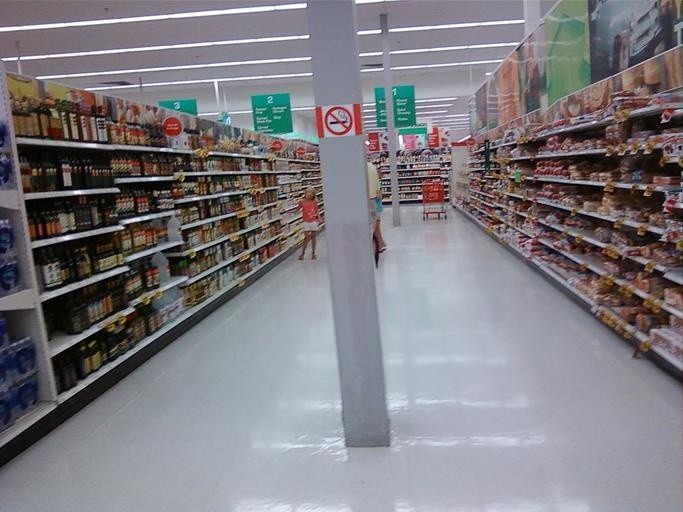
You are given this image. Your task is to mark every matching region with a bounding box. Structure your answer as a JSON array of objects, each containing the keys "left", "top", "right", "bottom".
[
  {"left": 367, "top": 161, "right": 387, "bottom": 253},
  {"left": 286, "top": 187, "right": 321, "bottom": 259}
]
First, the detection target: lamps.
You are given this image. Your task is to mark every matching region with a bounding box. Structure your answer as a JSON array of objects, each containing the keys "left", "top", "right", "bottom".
[{"left": 0, "top": 0, "right": 525, "bottom": 132}]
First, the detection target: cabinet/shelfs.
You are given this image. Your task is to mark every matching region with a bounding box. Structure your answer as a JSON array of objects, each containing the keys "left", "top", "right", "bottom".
[
  {"left": 0, "top": 65, "right": 328, "bottom": 471},
  {"left": 452, "top": 44, "right": 681, "bottom": 391},
  {"left": 366, "top": 149, "right": 451, "bottom": 204}
]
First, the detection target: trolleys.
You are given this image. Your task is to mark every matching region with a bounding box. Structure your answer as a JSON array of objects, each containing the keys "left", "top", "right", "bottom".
[{"left": 419, "top": 177, "right": 449, "bottom": 222}]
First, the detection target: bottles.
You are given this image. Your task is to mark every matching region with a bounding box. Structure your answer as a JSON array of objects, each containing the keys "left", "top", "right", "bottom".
[
  {"left": 374, "top": 154, "right": 452, "bottom": 202},
  {"left": 0, "top": 68, "right": 280, "bottom": 432}
]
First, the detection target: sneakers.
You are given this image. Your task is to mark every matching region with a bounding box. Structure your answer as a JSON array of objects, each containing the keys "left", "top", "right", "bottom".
[
  {"left": 299, "top": 255, "right": 317, "bottom": 260},
  {"left": 378, "top": 246, "right": 387, "bottom": 252}
]
